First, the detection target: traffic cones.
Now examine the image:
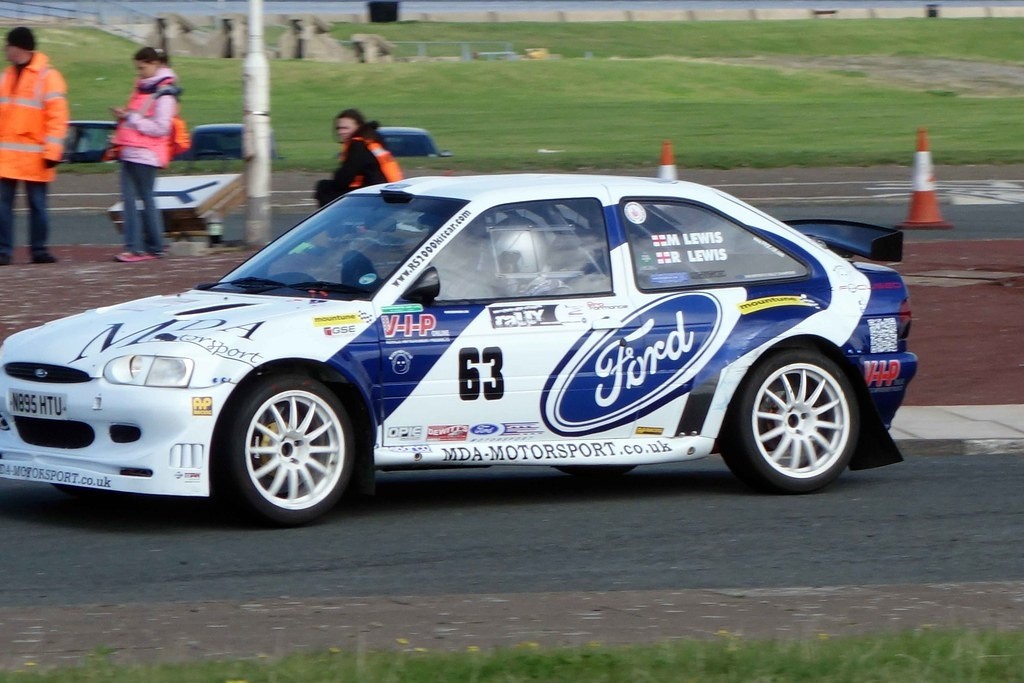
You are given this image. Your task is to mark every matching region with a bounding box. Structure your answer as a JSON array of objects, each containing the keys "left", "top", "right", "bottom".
[
  {"left": 895, "top": 129, "right": 956, "bottom": 229},
  {"left": 659, "top": 138, "right": 679, "bottom": 179}
]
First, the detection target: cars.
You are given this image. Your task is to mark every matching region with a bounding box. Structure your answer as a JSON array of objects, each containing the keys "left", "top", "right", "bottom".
[
  {"left": 0, "top": 174, "right": 918, "bottom": 527},
  {"left": 375, "top": 123, "right": 453, "bottom": 158},
  {"left": 173, "top": 122, "right": 287, "bottom": 160},
  {"left": 62, "top": 120, "right": 120, "bottom": 164}
]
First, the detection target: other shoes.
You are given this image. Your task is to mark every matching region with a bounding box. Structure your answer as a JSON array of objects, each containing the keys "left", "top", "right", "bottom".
[
  {"left": 0, "top": 254, "right": 13, "bottom": 266},
  {"left": 113, "top": 249, "right": 168, "bottom": 263},
  {"left": 29, "top": 252, "right": 57, "bottom": 263}
]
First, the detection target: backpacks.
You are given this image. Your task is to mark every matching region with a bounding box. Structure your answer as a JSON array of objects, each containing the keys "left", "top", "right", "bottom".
[{"left": 150, "top": 86, "right": 192, "bottom": 162}]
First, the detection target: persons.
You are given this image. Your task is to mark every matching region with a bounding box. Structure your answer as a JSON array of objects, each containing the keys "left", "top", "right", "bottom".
[
  {"left": 446, "top": 224, "right": 573, "bottom": 294},
  {"left": 110, "top": 47, "right": 181, "bottom": 261},
  {"left": 315, "top": 109, "right": 405, "bottom": 237},
  {"left": 0, "top": 27, "right": 68, "bottom": 264}
]
